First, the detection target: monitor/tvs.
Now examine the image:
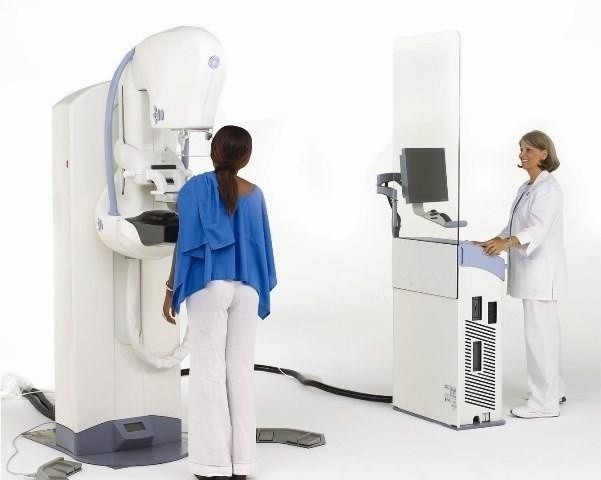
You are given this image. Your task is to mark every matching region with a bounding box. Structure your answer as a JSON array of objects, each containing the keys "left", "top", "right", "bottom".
[{"left": 401, "top": 148, "right": 449, "bottom": 203}]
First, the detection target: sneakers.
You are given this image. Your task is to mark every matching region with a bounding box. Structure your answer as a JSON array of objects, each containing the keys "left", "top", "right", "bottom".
[
  {"left": 508, "top": 389, "right": 568, "bottom": 419},
  {"left": 193, "top": 468, "right": 247, "bottom": 480}
]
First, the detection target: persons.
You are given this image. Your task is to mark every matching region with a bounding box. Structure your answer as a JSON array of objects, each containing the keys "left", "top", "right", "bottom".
[
  {"left": 162, "top": 125, "right": 280, "bottom": 480},
  {"left": 473, "top": 128, "right": 567, "bottom": 418}
]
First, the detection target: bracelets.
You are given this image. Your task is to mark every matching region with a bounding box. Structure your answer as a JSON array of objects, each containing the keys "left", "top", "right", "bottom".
[
  {"left": 507, "top": 237, "right": 513, "bottom": 248},
  {"left": 164, "top": 279, "right": 174, "bottom": 293}
]
